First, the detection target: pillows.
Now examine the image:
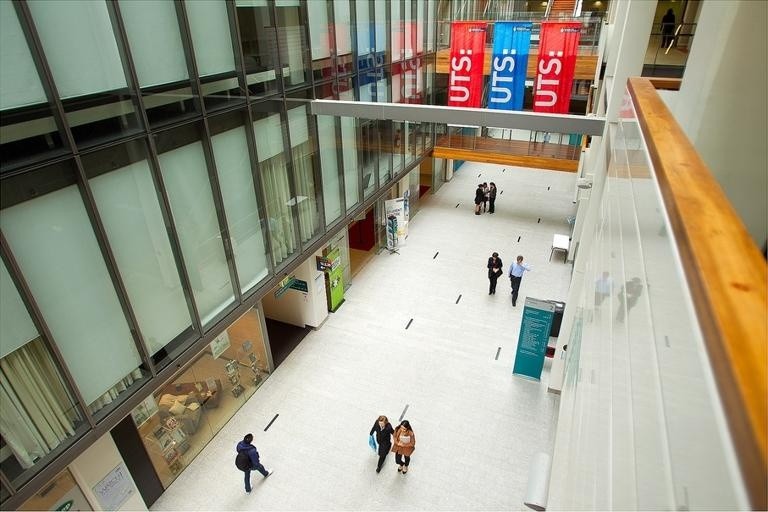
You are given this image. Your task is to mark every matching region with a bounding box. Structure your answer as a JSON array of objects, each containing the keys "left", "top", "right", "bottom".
[{"left": 169, "top": 400, "right": 186, "bottom": 416}]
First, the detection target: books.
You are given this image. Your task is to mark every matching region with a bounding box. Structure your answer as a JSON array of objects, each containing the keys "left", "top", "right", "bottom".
[{"left": 399, "top": 435, "right": 411, "bottom": 444}]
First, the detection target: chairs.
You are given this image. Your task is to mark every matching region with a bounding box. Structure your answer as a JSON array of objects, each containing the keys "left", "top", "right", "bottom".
[{"left": 158, "top": 396, "right": 201, "bottom": 434}]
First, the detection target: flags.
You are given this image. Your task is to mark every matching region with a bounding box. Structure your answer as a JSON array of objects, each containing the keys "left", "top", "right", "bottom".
[
  {"left": 389, "top": 19, "right": 423, "bottom": 126},
  {"left": 446, "top": 21, "right": 488, "bottom": 128},
  {"left": 532, "top": 22, "right": 583, "bottom": 114},
  {"left": 353, "top": 21, "right": 388, "bottom": 104},
  {"left": 319, "top": 22, "right": 352, "bottom": 101},
  {"left": 485, "top": 22, "right": 533, "bottom": 131}
]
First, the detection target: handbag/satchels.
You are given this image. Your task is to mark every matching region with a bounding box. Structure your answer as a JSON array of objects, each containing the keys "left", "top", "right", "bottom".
[{"left": 368, "top": 435, "right": 377, "bottom": 449}]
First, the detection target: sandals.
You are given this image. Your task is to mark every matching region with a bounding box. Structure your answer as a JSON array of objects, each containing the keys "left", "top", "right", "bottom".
[{"left": 398, "top": 462, "right": 409, "bottom": 474}]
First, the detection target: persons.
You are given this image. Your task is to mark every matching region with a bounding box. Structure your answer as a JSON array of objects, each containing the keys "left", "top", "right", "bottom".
[
  {"left": 235, "top": 434, "right": 275, "bottom": 494},
  {"left": 543, "top": 132, "right": 551, "bottom": 143},
  {"left": 391, "top": 419, "right": 416, "bottom": 474},
  {"left": 616, "top": 276, "right": 644, "bottom": 324},
  {"left": 487, "top": 252, "right": 503, "bottom": 295},
  {"left": 589, "top": 271, "right": 619, "bottom": 323},
  {"left": 508, "top": 255, "right": 531, "bottom": 306},
  {"left": 474, "top": 182, "right": 497, "bottom": 215},
  {"left": 660, "top": 8, "right": 676, "bottom": 49},
  {"left": 280, "top": 217, "right": 292, "bottom": 255},
  {"left": 370, "top": 415, "right": 394, "bottom": 474},
  {"left": 259, "top": 213, "right": 282, "bottom": 254}
]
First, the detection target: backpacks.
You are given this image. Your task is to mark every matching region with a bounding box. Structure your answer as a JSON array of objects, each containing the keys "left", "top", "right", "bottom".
[{"left": 235, "top": 446, "right": 256, "bottom": 472}]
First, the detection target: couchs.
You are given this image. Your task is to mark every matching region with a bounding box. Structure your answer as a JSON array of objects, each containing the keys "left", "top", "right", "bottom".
[{"left": 164, "top": 379, "right": 222, "bottom": 409}]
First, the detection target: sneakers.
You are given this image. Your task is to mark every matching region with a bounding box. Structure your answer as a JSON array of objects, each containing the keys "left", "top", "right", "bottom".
[
  {"left": 246, "top": 484, "right": 254, "bottom": 495},
  {"left": 376, "top": 467, "right": 381, "bottom": 473},
  {"left": 264, "top": 470, "right": 272, "bottom": 478}
]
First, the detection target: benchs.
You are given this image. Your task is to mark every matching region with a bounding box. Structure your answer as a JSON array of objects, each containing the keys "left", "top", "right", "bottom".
[{"left": 549, "top": 233, "right": 570, "bottom": 264}]
